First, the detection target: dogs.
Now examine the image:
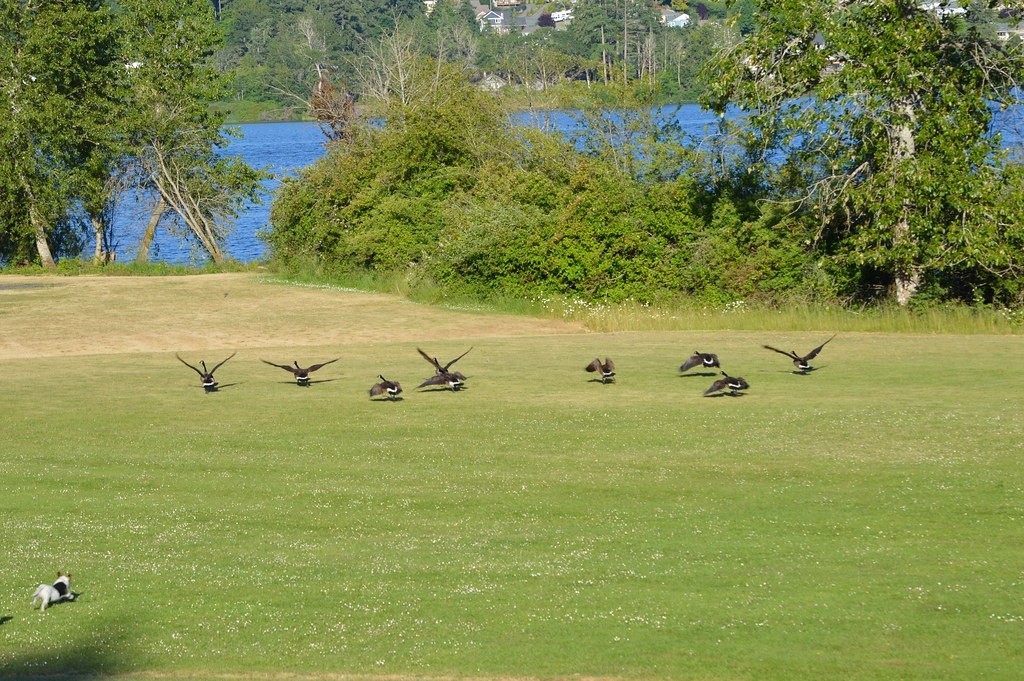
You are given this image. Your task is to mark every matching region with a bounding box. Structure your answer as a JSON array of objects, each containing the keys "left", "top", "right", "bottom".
[{"left": 30, "top": 571, "right": 74, "bottom": 612}]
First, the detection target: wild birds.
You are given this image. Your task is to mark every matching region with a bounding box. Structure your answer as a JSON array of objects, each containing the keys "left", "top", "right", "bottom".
[
  {"left": 762, "top": 333, "right": 836, "bottom": 373},
  {"left": 679, "top": 351, "right": 721, "bottom": 371},
  {"left": 260, "top": 357, "right": 339, "bottom": 386},
  {"left": 414, "top": 347, "right": 474, "bottom": 392},
  {"left": 704, "top": 371, "right": 749, "bottom": 396},
  {"left": 585, "top": 357, "right": 617, "bottom": 384},
  {"left": 370, "top": 374, "right": 402, "bottom": 399},
  {"left": 175, "top": 352, "right": 236, "bottom": 394}
]
